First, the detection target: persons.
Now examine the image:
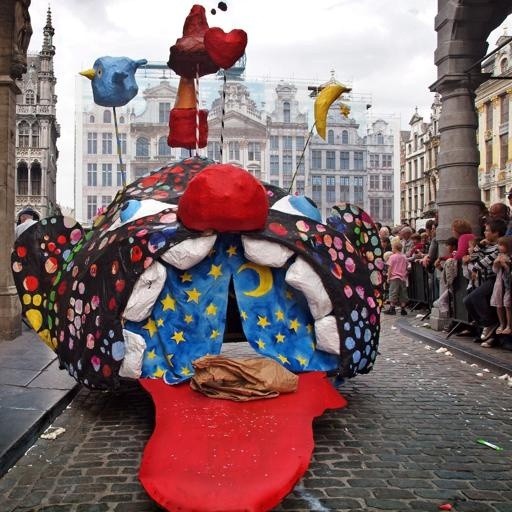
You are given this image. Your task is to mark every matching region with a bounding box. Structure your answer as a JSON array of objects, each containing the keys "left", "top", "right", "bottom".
[
  {"left": 374, "top": 187, "right": 511, "bottom": 353},
  {"left": 16, "top": 207, "right": 40, "bottom": 225}
]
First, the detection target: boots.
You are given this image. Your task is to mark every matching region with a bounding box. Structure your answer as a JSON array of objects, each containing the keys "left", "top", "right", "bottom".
[{"left": 384, "top": 306, "right": 407, "bottom": 315}]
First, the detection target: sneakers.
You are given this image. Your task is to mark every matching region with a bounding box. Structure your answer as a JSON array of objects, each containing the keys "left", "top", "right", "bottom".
[{"left": 457, "top": 320, "right": 512, "bottom": 347}]
